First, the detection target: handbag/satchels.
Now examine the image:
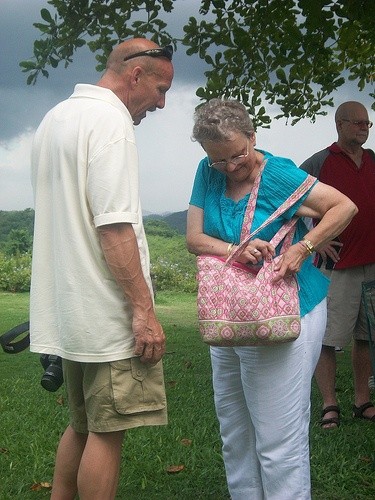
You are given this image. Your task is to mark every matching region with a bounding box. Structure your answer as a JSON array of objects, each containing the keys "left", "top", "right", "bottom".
[{"left": 197, "top": 158, "right": 318, "bottom": 347}]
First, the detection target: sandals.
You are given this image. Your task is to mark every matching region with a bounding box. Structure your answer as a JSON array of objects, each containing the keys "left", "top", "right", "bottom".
[
  {"left": 321, "top": 405, "right": 341, "bottom": 428},
  {"left": 352, "top": 401, "right": 375, "bottom": 421}
]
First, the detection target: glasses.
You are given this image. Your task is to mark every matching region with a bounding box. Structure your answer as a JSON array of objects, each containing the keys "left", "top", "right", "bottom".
[
  {"left": 342, "top": 117, "right": 374, "bottom": 129},
  {"left": 208, "top": 133, "right": 252, "bottom": 169},
  {"left": 123, "top": 46, "right": 173, "bottom": 62}
]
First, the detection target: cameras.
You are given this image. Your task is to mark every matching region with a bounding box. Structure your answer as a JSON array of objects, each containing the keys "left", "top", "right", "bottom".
[{"left": 40, "top": 354, "right": 65, "bottom": 392}]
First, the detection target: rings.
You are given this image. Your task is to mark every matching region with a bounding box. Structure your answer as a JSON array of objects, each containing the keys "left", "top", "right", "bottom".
[{"left": 253, "top": 249, "right": 259, "bottom": 254}]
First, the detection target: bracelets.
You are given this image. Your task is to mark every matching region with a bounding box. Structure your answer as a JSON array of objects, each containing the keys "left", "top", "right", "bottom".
[
  {"left": 298, "top": 237, "right": 314, "bottom": 256},
  {"left": 227, "top": 242, "right": 238, "bottom": 259}
]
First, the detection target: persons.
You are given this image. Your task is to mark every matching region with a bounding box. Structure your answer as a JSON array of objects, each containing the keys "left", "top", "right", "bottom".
[
  {"left": 298, "top": 102, "right": 375, "bottom": 430},
  {"left": 184, "top": 99, "right": 359, "bottom": 500},
  {"left": 31, "top": 38, "right": 175, "bottom": 500}
]
